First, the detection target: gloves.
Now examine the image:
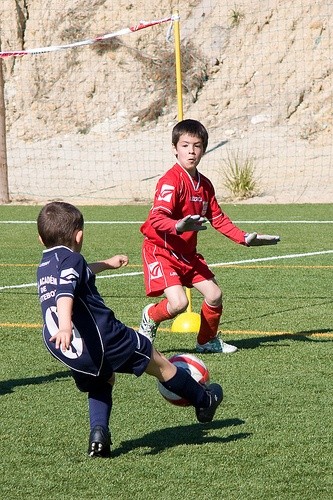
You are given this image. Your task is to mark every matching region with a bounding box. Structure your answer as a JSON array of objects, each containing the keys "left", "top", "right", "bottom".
[
  {"left": 246, "top": 232, "right": 279, "bottom": 246},
  {"left": 176, "top": 215, "right": 207, "bottom": 231}
]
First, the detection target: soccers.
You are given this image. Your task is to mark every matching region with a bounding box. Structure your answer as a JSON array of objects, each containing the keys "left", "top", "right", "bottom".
[{"left": 157, "top": 352, "right": 209, "bottom": 408}]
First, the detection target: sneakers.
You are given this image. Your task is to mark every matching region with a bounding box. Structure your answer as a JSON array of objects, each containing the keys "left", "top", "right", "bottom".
[
  {"left": 196, "top": 383, "right": 223, "bottom": 422},
  {"left": 138, "top": 303, "right": 157, "bottom": 344},
  {"left": 197, "top": 337, "right": 236, "bottom": 353},
  {"left": 88, "top": 427, "right": 110, "bottom": 455}
]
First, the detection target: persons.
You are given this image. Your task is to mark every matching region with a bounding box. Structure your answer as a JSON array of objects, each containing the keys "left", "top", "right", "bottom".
[
  {"left": 37, "top": 201, "right": 224, "bottom": 456},
  {"left": 138, "top": 120, "right": 281, "bottom": 354}
]
